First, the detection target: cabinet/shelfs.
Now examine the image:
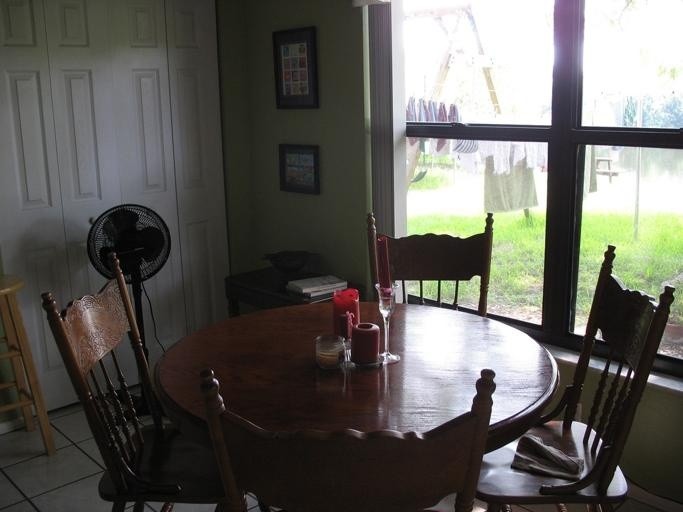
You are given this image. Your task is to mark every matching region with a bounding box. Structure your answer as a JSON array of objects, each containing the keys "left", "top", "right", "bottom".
[{"left": 0, "top": 0, "right": 230, "bottom": 417}]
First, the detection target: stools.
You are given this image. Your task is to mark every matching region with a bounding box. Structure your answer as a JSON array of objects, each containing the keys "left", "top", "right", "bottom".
[{"left": 0, "top": 274, "right": 56, "bottom": 456}]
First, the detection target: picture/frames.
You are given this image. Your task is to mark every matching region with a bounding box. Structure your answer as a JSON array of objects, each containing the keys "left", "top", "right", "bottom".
[
  {"left": 277, "top": 143, "right": 320, "bottom": 196},
  {"left": 271, "top": 25, "right": 320, "bottom": 111}
]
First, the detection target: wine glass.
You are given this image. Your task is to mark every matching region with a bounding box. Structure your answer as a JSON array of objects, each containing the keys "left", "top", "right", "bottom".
[{"left": 374, "top": 283, "right": 402, "bottom": 365}]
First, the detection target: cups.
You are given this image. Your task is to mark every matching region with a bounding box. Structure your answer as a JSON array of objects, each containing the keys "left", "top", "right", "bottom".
[
  {"left": 315, "top": 334, "right": 345, "bottom": 369},
  {"left": 334, "top": 288, "right": 360, "bottom": 340}
]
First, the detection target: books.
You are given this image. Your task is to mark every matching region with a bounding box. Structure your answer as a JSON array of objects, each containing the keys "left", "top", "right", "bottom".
[{"left": 286, "top": 276, "right": 348, "bottom": 298}]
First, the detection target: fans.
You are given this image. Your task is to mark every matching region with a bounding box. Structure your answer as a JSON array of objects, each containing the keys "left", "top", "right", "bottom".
[{"left": 86, "top": 203, "right": 172, "bottom": 426}]
